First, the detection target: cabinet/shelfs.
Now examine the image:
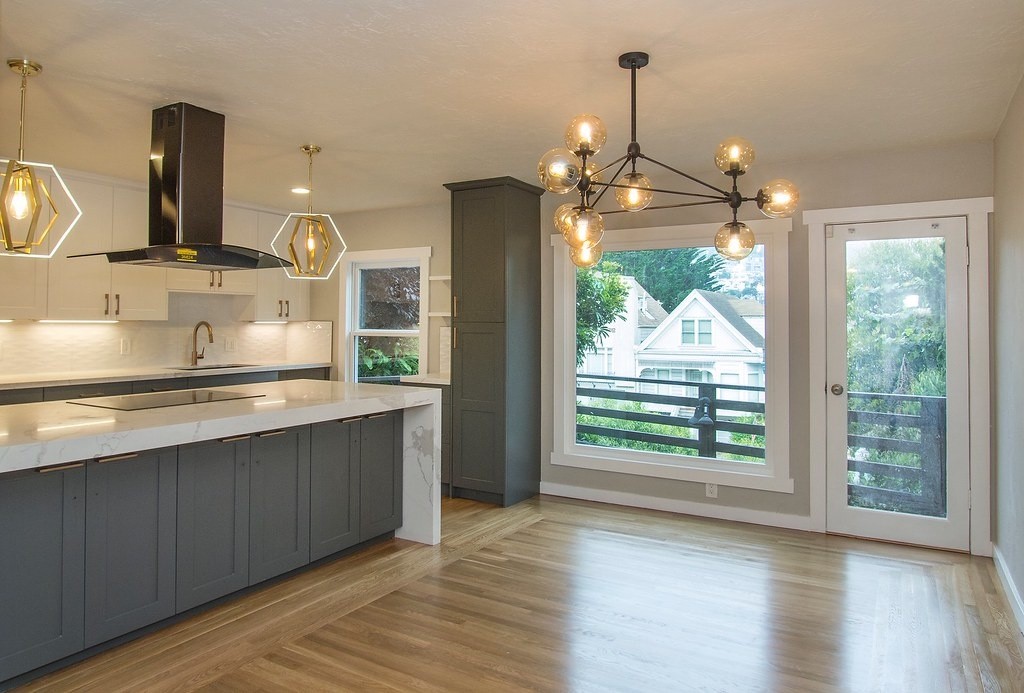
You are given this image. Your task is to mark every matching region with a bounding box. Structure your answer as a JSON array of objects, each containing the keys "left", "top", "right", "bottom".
[
  {"left": 234, "top": 213, "right": 326, "bottom": 322},
  {"left": 441, "top": 175, "right": 545, "bottom": 507},
  {"left": 168, "top": 202, "right": 258, "bottom": 295},
  {"left": 0, "top": 160, "right": 50, "bottom": 321},
  {"left": 1, "top": 448, "right": 179, "bottom": 685},
  {"left": 50, "top": 167, "right": 169, "bottom": 321},
  {"left": 174, "top": 423, "right": 312, "bottom": 616},
  {"left": 309, "top": 409, "right": 406, "bottom": 564},
  {"left": 0, "top": 368, "right": 331, "bottom": 406}
]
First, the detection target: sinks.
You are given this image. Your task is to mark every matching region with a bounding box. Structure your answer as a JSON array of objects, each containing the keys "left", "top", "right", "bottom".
[{"left": 162, "top": 363, "right": 261, "bottom": 371}]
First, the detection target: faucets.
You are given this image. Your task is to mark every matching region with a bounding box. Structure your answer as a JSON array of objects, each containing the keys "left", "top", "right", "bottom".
[{"left": 190, "top": 321, "right": 214, "bottom": 366}]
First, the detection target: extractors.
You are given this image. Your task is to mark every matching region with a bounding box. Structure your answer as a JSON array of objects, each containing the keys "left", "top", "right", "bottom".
[{"left": 103, "top": 102, "right": 295, "bottom": 273}]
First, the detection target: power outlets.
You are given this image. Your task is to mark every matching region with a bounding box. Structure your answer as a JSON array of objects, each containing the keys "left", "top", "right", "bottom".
[
  {"left": 225, "top": 337, "right": 239, "bottom": 352},
  {"left": 121, "top": 338, "right": 132, "bottom": 355}
]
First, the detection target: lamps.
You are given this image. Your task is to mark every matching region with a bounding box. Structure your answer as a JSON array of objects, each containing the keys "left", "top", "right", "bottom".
[
  {"left": 270, "top": 143, "right": 347, "bottom": 280},
  {"left": 0, "top": 58, "right": 82, "bottom": 259},
  {"left": 537, "top": 49, "right": 799, "bottom": 267},
  {"left": 688, "top": 395, "right": 714, "bottom": 429}
]
